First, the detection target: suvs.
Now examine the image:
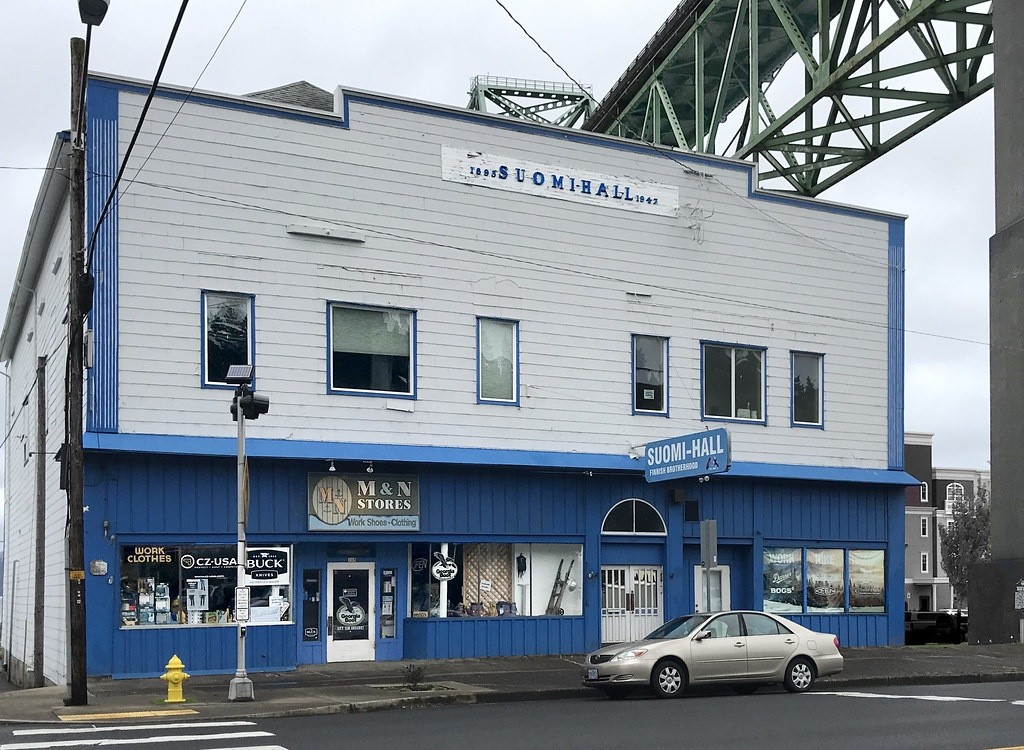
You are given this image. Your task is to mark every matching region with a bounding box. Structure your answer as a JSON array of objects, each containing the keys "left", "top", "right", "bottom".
[{"left": 904, "top": 611, "right": 957, "bottom": 633}]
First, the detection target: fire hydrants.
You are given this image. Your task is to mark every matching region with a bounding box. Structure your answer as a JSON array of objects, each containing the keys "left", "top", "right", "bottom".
[{"left": 159, "top": 654, "right": 192, "bottom": 704}]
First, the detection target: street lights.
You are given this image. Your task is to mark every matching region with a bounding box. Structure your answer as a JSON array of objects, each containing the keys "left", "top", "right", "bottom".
[{"left": 226, "top": 365, "right": 269, "bottom": 699}]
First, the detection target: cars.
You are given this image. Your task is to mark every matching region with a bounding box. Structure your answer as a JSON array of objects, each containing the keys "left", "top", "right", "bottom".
[{"left": 579, "top": 609, "right": 844, "bottom": 699}]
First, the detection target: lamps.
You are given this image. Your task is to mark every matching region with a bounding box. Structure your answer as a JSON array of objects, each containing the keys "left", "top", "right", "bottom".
[
  {"left": 329, "top": 462, "right": 336, "bottom": 471},
  {"left": 516, "top": 552, "right": 526, "bottom": 572},
  {"left": 367, "top": 462, "right": 373, "bottom": 472}
]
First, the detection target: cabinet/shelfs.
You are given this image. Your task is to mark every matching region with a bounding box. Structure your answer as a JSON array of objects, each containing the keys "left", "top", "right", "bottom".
[{"left": 137, "top": 576, "right": 210, "bottom": 624}]
